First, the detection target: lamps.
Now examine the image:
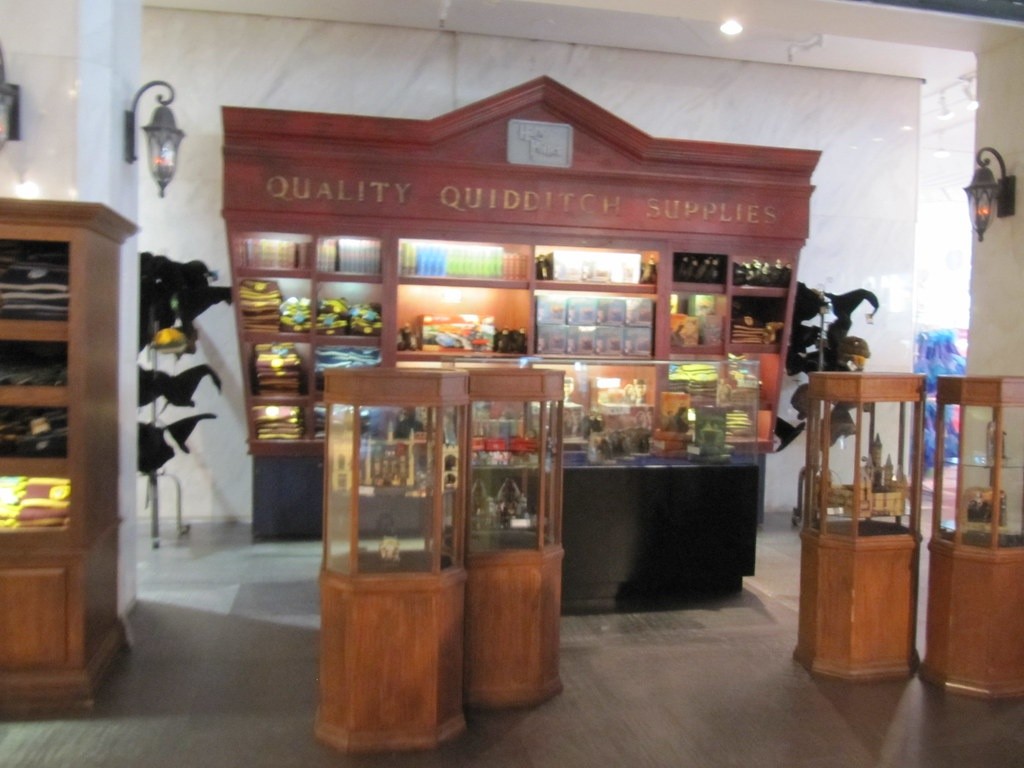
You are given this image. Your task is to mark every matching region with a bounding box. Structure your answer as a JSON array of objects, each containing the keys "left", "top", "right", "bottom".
[
  {"left": 962, "top": 148, "right": 1014, "bottom": 242},
  {"left": 123, "top": 80, "right": 185, "bottom": 197},
  {"left": 0, "top": 50, "right": 20, "bottom": 153}
]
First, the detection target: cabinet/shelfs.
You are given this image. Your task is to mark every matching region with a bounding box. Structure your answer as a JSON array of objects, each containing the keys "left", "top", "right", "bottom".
[
  {"left": 0, "top": 195, "right": 139, "bottom": 721},
  {"left": 314, "top": 367, "right": 470, "bottom": 752},
  {"left": 230, "top": 227, "right": 801, "bottom": 543},
  {"left": 793, "top": 371, "right": 924, "bottom": 680},
  {"left": 459, "top": 367, "right": 565, "bottom": 710},
  {"left": 915, "top": 376, "right": 1024, "bottom": 697}
]
